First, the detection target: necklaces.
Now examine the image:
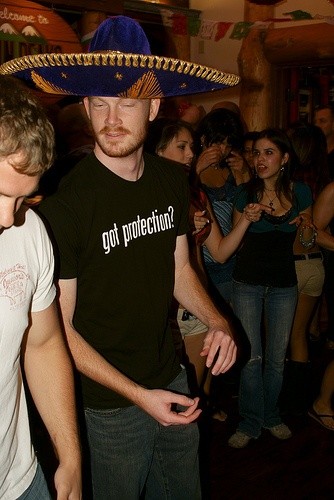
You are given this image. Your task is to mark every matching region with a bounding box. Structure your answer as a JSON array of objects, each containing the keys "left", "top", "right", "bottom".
[{"left": 264, "top": 187, "right": 280, "bottom": 206}]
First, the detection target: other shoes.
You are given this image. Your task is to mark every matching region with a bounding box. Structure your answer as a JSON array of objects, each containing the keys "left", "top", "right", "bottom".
[
  {"left": 265, "top": 423, "right": 293, "bottom": 439},
  {"left": 228, "top": 431, "right": 253, "bottom": 448},
  {"left": 200, "top": 397, "right": 227, "bottom": 424}
]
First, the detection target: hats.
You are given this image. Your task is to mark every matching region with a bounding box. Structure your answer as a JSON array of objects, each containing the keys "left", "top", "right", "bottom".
[{"left": 0, "top": 15, "right": 240, "bottom": 99}]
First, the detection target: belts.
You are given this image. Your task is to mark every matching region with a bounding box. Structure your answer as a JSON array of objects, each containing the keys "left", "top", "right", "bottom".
[{"left": 293, "top": 252, "right": 322, "bottom": 260}]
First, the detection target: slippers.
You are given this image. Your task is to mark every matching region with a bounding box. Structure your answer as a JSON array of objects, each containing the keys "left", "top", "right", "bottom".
[{"left": 308, "top": 407, "right": 334, "bottom": 430}]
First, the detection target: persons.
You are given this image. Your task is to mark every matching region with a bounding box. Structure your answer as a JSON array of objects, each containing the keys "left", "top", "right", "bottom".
[
  {"left": 0, "top": 74, "right": 85, "bottom": 500},
  {"left": 193, "top": 100, "right": 334, "bottom": 431},
  {"left": 0, "top": 16, "right": 242, "bottom": 500},
  {"left": 148, "top": 120, "right": 274, "bottom": 423},
  {"left": 227, "top": 127, "right": 315, "bottom": 450}
]
